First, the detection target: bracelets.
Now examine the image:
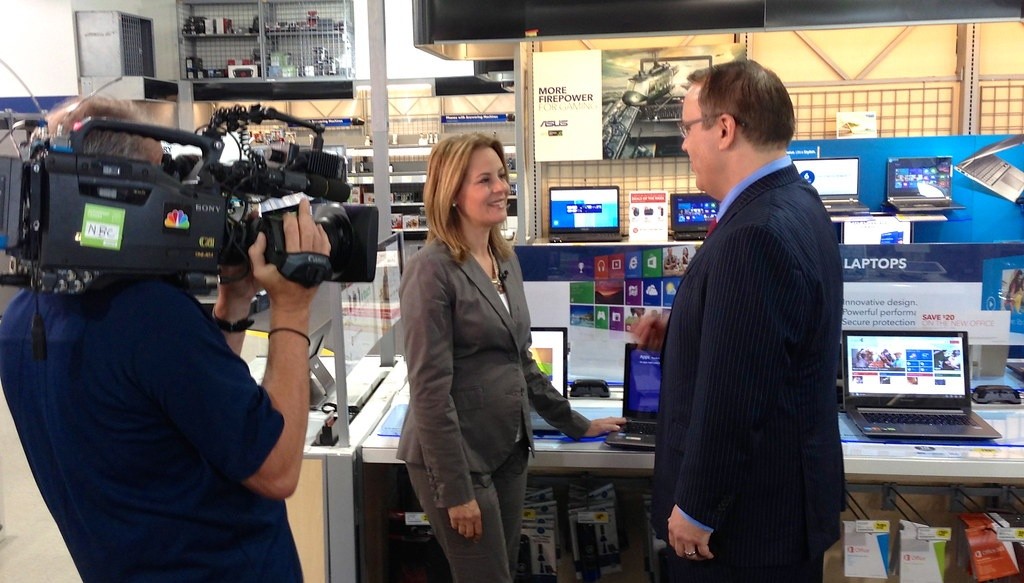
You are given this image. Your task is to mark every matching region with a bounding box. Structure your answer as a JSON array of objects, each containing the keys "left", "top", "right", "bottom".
[{"left": 267, "top": 327, "right": 311, "bottom": 348}]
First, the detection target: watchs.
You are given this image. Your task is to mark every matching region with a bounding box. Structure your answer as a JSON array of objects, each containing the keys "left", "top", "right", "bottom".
[{"left": 211, "top": 307, "right": 255, "bottom": 333}]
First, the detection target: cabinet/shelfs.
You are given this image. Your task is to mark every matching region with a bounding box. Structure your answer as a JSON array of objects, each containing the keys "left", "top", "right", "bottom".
[
  {"left": 174, "top": 0, "right": 357, "bottom": 84},
  {"left": 353, "top": 142, "right": 519, "bottom": 241}
]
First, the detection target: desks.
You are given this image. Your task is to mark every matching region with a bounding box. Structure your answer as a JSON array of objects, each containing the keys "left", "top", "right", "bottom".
[
  {"left": 881, "top": 204, "right": 973, "bottom": 243},
  {"left": 360, "top": 397, "right": 1024, "bottom": 487},
  {"left": 830, "top": 213, "right": 875, "bottom": 244}
]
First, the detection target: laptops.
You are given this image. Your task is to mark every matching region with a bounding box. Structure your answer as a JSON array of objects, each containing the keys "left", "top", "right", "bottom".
[
  {"left": 670, "top": 193, "right": 721, "bottom": 241},
  {"left": 548, "top": 186, "right": 622, "bottom": 243},
  {"left": 841, "top": 330, "right": 1002, "bottom": 440},
  {"left": 792, "top": 157, "right": 870, "bottom": 212},
  {"left": 525, "top": 326, "right": 567, "bottom": 436},
  {"left": 887, "top": 157, "right": 965, "bottom": 211},
  {"left": 954, "top": 135, "right": 1024, "bottom": 202},
  {"left": 607, "top": 343, "right": 662, "bottom": 452}
]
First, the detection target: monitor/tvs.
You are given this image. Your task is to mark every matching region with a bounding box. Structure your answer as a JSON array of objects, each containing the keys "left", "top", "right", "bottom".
[{"left": 841, "top": 212, "right": 914, "bottom": 245}]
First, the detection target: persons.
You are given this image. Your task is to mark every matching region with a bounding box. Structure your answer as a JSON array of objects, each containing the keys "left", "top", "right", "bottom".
[
  {"left": 1003, "top": 270, "right": 1024, "bottom": 306},
  {"left": 394, "top": 131, "right": 628, "bottom": 583},
  {"left": 631, "top": 58, "right": 846, "bottom": 583},
  {"left": 0, "top": 94, "right": 331, "bottom": 583},
  {"left": 663, "top": 247, "right": 690, "bottom": 273}
]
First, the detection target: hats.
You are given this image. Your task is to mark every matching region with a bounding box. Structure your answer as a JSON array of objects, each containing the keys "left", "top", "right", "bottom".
[{"left": 894, "top": 352, "right": 902, "bottom": 355}]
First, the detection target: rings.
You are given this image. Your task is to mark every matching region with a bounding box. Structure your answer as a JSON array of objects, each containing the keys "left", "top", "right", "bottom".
[{"left": 685, "top": 551, "right": 696, "bottom": 556}]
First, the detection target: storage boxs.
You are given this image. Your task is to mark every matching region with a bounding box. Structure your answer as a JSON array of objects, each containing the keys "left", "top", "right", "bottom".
[
  {"left": 215, "top": 17, "right": 233, "bottom": 34},
  {"left": 205, "top": 18, "right": 217, "bottom": 34},
  {"left": 228, "top": 64, "right": 258, "bottom": 78},
  {"left": 517, "top": 483, "right": 1021, "bottom": 583}
]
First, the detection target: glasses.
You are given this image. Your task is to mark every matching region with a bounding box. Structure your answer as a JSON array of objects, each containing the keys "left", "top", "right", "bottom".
[{"left": 676, "top": 113, "right": 747, "bottom": 140}]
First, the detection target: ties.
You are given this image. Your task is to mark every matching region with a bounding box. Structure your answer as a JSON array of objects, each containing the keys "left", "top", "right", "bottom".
[{"left": 704, "top": 218, "right": 718, "bottom": 238}]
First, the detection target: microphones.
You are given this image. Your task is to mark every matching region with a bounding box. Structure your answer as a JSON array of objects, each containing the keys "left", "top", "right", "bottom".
[{"left": 305, "top": 175, "right": 350, "bottom": 202}]
